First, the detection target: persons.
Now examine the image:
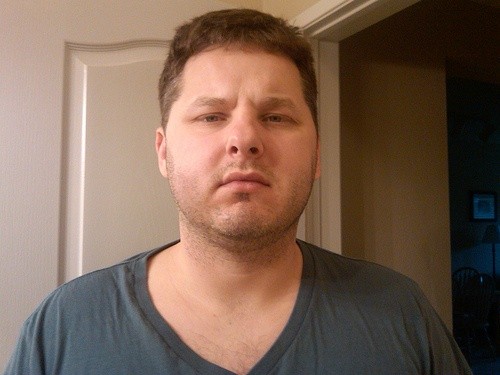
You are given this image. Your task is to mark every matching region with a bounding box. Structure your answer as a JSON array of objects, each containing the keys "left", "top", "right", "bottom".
[{"left": 3, "top": 9, "right": 473, "bottom": 375}]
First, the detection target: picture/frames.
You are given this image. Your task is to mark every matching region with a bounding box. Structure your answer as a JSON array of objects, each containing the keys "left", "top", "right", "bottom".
[{"left": 470, "top": 190, "right": 497, "bottom": 222}]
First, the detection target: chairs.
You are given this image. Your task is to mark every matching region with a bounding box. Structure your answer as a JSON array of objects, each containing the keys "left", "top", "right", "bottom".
[
  {"left": 454, "top": 274, "right": 496, "bottom": 366},
  {"left": 452, "top": 266, "right": 480, "bottom": 308}
]
